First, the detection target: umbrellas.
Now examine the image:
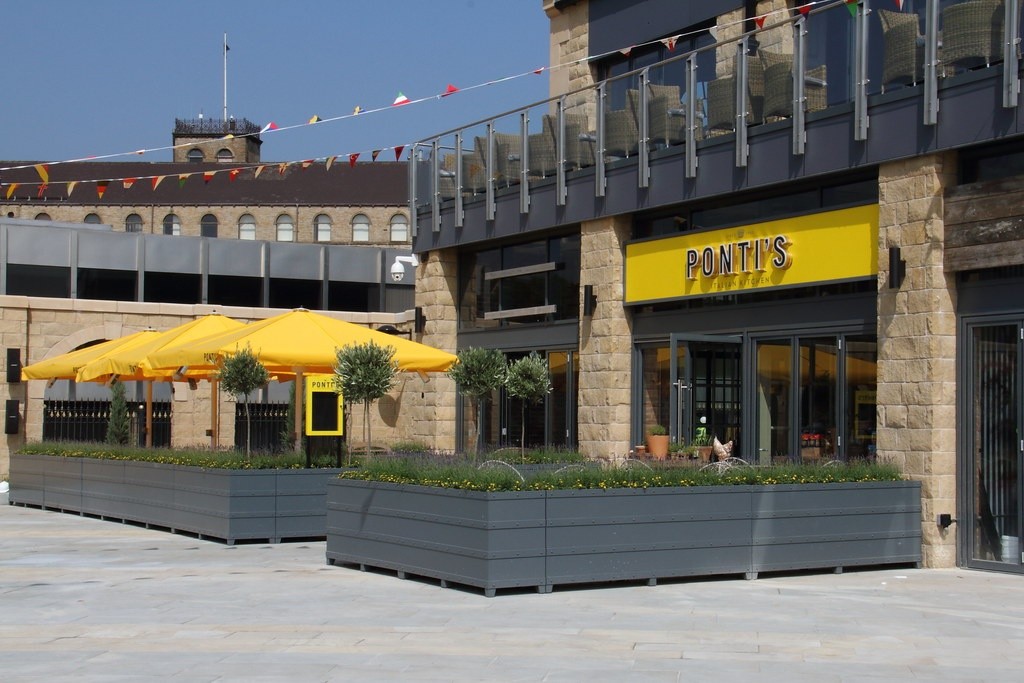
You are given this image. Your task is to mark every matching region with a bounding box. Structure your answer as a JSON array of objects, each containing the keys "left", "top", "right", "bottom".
[
  {"left": 75, "top": 310, "right": 295, "bottom": 449},
  {"left": 149, "top": 308, "right": 463, "bottom": 465},
  {"left": 21, "top": 326, "right": 167, "bottom": 448}
]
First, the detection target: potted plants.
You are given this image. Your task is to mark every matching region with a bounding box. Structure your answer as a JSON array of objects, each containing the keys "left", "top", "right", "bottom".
[
  {"left": 687, "top": 427, "right": 713, "bottom": 462},
  {"left": 646, "top": 425, "right": 679, "bottom": 458}
]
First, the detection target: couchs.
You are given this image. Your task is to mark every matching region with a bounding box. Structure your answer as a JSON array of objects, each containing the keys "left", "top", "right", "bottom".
[
  {"left": 877, "top": 9, "right": 955, "bottom": 94},
  {"left": 758, "top": 49, "right": 827, "bottom": 125},
  {"left": 528, "top": 114, "right": 557, "bottom": 179},
  {"left": 942, "top": 0, "right": 1020, "bottom": 78},
  {"left": 565, "top": 114, "right": 596, "bottom": 170},
  {"left": 707, "top": 55, "right": 764, "bottom": 129},
  {"left": 647, "top": 86, "right": 705, "bottom": 148},
  {"left": 439, "top": 153, "right": 455, "bottom": 198},
  {"left": 605, "top": 89, "right": 639, "bottom": 159},
  {"left": 462, "top": 136, "right": 486, "bottom": 196}
]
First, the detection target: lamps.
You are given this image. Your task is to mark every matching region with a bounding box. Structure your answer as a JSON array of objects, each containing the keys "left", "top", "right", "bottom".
[
  {"left": 376, "top": 325, "right": 412, "bottom": 341},
  {"left": 888, "top": 247, "right": 907, "bottom": 289},
  {"left": 415, "top": 306, "right": 426, "bottom": 333},
  {"left": 584, "top": 284, "right": 597, "bottom": 317}
]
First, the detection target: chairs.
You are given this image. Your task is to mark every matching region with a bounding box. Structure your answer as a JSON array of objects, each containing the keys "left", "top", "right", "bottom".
[{"left": 495, "top": 134, "right": 521, "bottom": 190}]
